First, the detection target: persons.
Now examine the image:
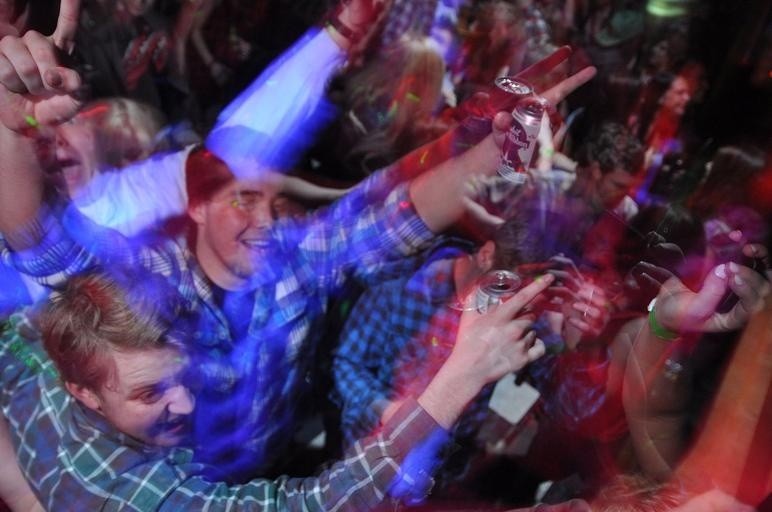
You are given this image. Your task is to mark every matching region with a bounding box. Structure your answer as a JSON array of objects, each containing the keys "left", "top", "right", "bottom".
[{"left": 0, "top": 0, "right": 770, "bottom": 511}]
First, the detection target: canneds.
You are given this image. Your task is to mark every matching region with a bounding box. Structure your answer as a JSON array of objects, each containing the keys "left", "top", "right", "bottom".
[
  {"left": 496, "top": 96, "right": 545, "bottom": 186},
  {"left": 477, "top": 269, "right": 522, "bottom": 314},
  {"left": 491, "top": 76, "right": 536, "bottom": 114}
]
